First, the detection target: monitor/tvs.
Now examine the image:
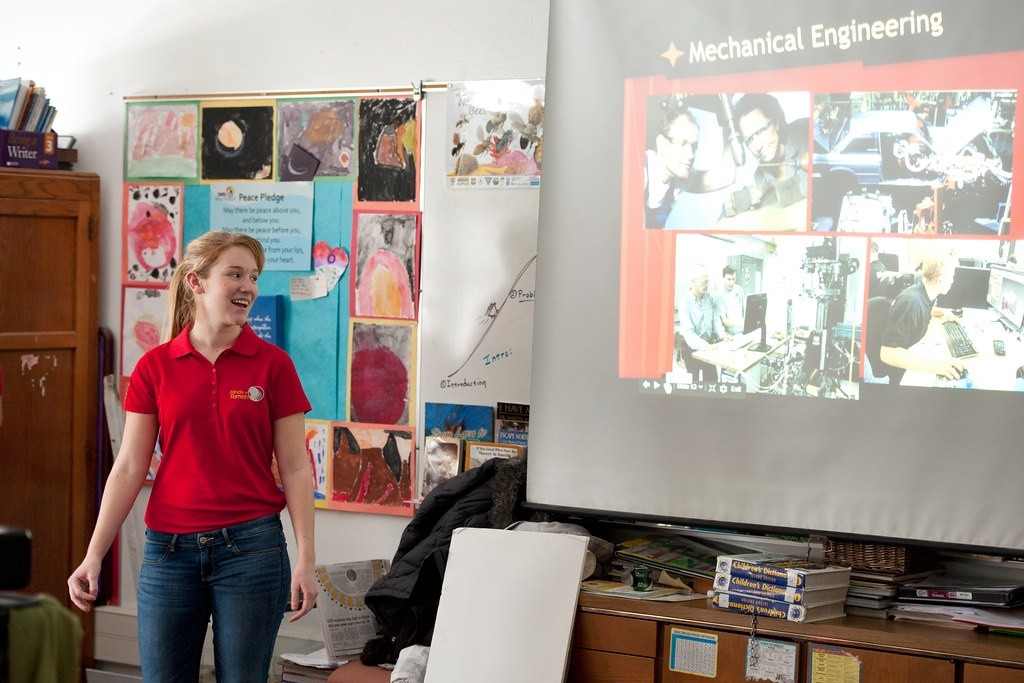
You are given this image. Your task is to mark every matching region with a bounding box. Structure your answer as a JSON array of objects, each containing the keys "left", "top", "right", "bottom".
[
  {"left": 986, "top": 266, "right": 1024, "bottom": 332},
  {"left": 742, "top": 292, "right": 772, "bottom": 352}
]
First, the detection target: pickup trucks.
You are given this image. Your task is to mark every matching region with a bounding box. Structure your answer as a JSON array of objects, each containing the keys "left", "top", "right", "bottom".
[{"left": 808, "top": 94, "right": 1015, "bottom": 218}]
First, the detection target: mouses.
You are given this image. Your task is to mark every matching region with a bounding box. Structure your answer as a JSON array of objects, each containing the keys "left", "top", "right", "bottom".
[{"left": 937, "top": 366, "right": 968, "bottom": 380}]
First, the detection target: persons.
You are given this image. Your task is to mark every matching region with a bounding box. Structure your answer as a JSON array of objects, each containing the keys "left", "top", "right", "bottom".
[
  {"left": 712, "top": 266, "right": 747, "bottom": 336},
  {"left": 733, "top": 93, "right": 808, "bottom": 213},
  {"left": 813, "top": 98, "right": 826, "bottom": 121},
  {"left": 869, "top": 241, "right": 886, "bottom": 299},
  {"left": 679, "top": 267, "right": 734, "bottom": 383},
  {"left": 642, "top": 103, "right": 699, "bottom": 230},
  {"left": 67, "top": 230, "right": 318, "bottom": 683},
  {"left": 880, "top": 250, "right": 966, "bottom": 385}
]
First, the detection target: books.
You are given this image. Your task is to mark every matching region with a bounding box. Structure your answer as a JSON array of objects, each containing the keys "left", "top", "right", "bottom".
[
  {"left": 581, "top": 533, "right": 1024, "bottom": 636},
  {"left": 419, "top": 402, "right": 530, "bottom": 501},
  {"left": 0, "top": 77, "right": 58, "bottom": 132},
  {"left": 315, "top": 558, "right": 389, "bottom": 665}
]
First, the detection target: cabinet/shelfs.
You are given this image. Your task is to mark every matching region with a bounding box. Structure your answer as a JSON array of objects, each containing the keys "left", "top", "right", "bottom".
[
  {"left": 961, "top": 628, "right": 1023, "bottom": 683},
  {"left": 1, "top": 162, "right": 100, "bottom": 663},
  {"left": 561, "top": 593, "right": 659, "bottom": 683},
  {"left": 662, "top": 597, "right": 962, "bottom": 683}
]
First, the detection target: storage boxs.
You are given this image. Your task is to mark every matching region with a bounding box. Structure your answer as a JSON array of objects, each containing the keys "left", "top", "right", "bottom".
[{"left": 0, "top": 129, "right": 59, "bottom": 170}]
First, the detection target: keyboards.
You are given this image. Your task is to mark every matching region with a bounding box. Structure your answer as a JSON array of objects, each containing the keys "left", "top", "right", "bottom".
[{"left": 942, "top": 320, "right": 977, "bottom": 358}]
